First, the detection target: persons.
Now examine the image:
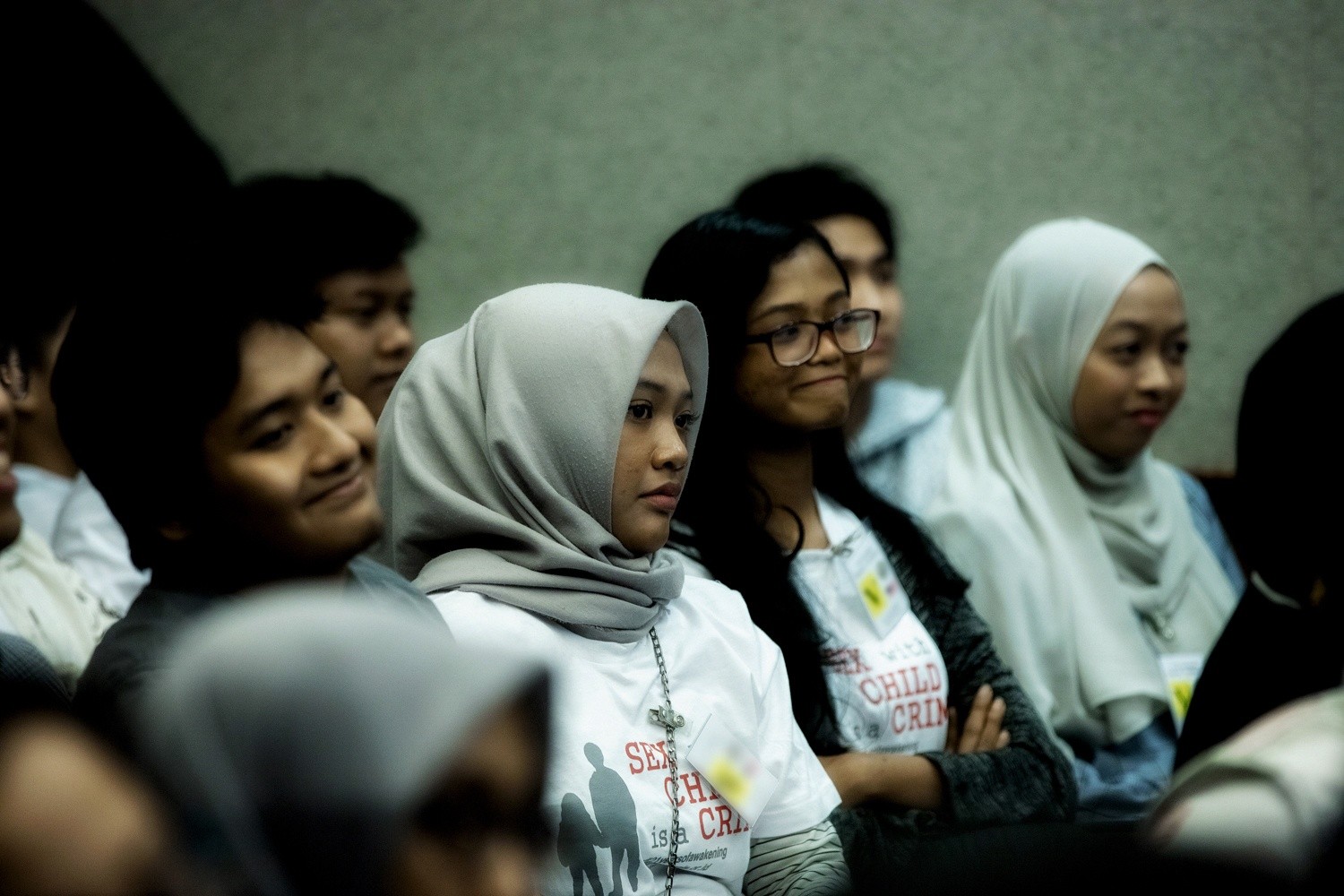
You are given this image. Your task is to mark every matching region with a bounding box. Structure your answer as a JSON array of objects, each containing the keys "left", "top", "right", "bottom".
[
  {"left": 896, "top": 216, "right": 1245, "bottom": 894},
  {"left": 728, "top": 157, "right": 944, "bottom": 504},
  {"left": 1137, "top": 289, "right": 1344, "bottom": 869},
  {"left": 227, "top": 168, "right": 428, "bottom": 424},
  {"left": 0, "top": 686, "right": 184, "bottom": 896},
  {"left": 375, "top": 280, "right": 850, "bottom": 896},
  {"left": 51, "top": 263, "right": 461, "bottom": 647},
  {"left": 0, "top": 243, "right": 152, "bottom": 681},
  {"left": 138, "top": 581, "right": 557, "bottom": 896},
  {"left": 642, "top": 209, "right": 1082, "bottom": 894}
]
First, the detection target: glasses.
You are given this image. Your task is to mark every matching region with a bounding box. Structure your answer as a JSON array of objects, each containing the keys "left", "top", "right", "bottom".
[{"left": 745, "top": 307, "right": 885, "bottom": 367}]
